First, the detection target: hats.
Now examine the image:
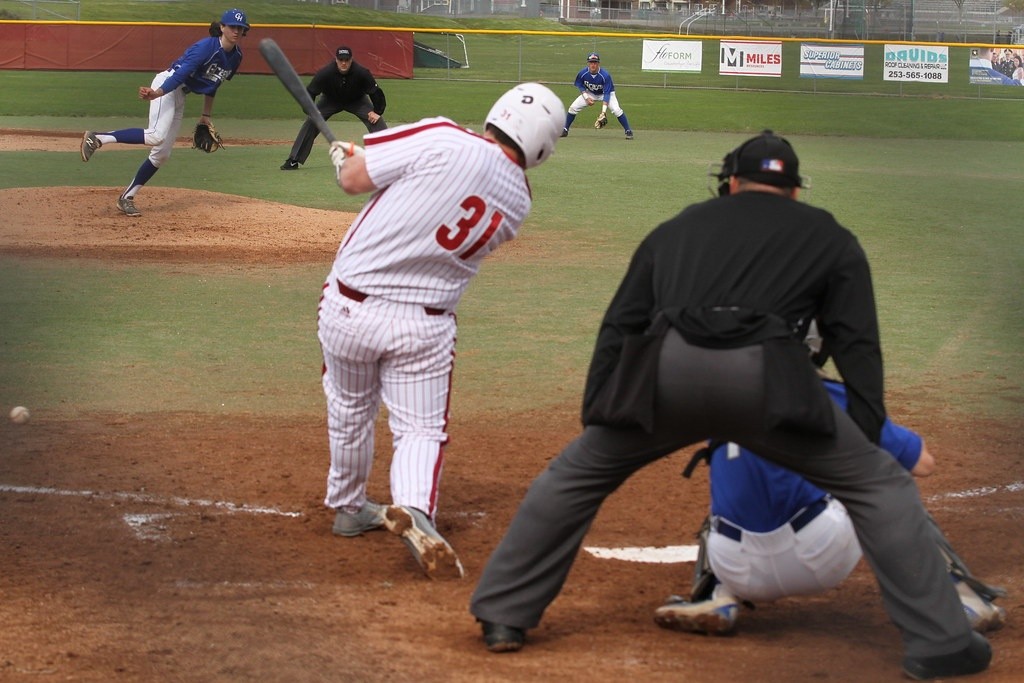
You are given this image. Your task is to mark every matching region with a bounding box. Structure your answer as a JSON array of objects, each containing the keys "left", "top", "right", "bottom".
[
  {"left": 336, "top": 47, "right": 352, "bottom": 60},
  {"left": 730, "top": 133, "right": 798, "bottom": 190},
  {"left": 220, "top": 9, "right": 250, "bottom": 31},
  {"left": 587, "top": 53, "right": 600, "bottom": 62}
]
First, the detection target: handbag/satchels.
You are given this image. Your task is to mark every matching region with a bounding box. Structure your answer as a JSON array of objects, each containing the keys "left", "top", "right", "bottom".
[
  {"left": 583, "top": 317, "right": 669, "bottom": 433},
  {"left": 761, "top": 336, "right": 837, "bottom": 441}
]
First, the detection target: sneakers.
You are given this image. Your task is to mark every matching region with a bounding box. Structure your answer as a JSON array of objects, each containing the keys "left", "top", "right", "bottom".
[
  {"left": 282, "top": 159, "right": 300, "bottom": 170},
  {"left": 478, "top": 620, "right": 524, "bottom": 650},
  {"left": 117, "top": 196, "right": 141, "bottom": 217},
  {"left": 954, "top": 581, "right": 1006, "bottom": 633},
  {"left": 625, "top": 130, "right": 633, "bottom": 139},
  {"left": 382, "top": 505, "right": 465, "bottom": 583},
  {"left": 560, "top": 129, "right": 568, "bottom": 137},
  {"left": 651, "top": 591, "right": 739, "bottom": 633},
  {"left": 81, "top": 130, "right": 104, "bottom": 163},
  {"left": 331, "top": 498, "right": 391, "bottom": 535},
  {"left": 900, "top": 630, "right": 993, "bottom": 679}
]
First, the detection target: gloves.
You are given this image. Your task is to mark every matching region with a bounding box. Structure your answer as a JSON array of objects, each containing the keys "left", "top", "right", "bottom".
[{"left": 329, "top": 140, "right": 369, "bottom": 180}]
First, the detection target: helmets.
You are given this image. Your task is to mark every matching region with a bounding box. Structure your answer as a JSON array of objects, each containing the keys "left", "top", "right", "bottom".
[{"left": 484, "top": 82, "right": 568, "bottom": 170}]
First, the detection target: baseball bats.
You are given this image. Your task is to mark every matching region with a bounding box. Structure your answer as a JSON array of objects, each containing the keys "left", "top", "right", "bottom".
[{"left": 257, "top": 37, "right": 337, "bottom": 146}]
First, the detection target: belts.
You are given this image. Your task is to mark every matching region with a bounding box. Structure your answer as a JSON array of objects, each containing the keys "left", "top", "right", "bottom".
[
  {"left": 338, "top": 278, "right": 449, "bottom": 317},
  {"left": 713, "top": 499, "right": 829, "bottom": 542},
  {"left": 168, "top": 68, "right": 191, "bottom": 94}
]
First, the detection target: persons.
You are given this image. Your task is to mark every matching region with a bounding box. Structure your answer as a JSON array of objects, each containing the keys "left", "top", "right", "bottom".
[
  {"left": 560, "top": 52, "right": 633, "bottom": 139},
  {"left": 281, "top": 48, "right": 388, "bottom": 170},
  {"left": 970, "top": 48, "right": 1024, "bottom": 84},
  {"left": 318, "top": 82, "right": 568, "bottom": 585},
  {"left": 81, "top": 8, "right": 250, "bottom": 216},
  {"left": 655, "top": 319, "right": 1004, "bottom": 635},
  {"left": 470, "top": 131, "right": 992, "bottom": 680}
]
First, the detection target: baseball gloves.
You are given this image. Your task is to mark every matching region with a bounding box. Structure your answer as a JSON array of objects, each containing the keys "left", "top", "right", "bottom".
[
  {"left": 190, "top": 122, "right": 226, "bottom": 155},
  {"left": 594, "top": 113, "right": 608, "bottom": 129}
]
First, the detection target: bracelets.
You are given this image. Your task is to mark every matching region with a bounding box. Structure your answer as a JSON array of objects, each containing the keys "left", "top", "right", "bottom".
[{"left": 202, "top": 113, "right": 210, "bottom": 117}]
